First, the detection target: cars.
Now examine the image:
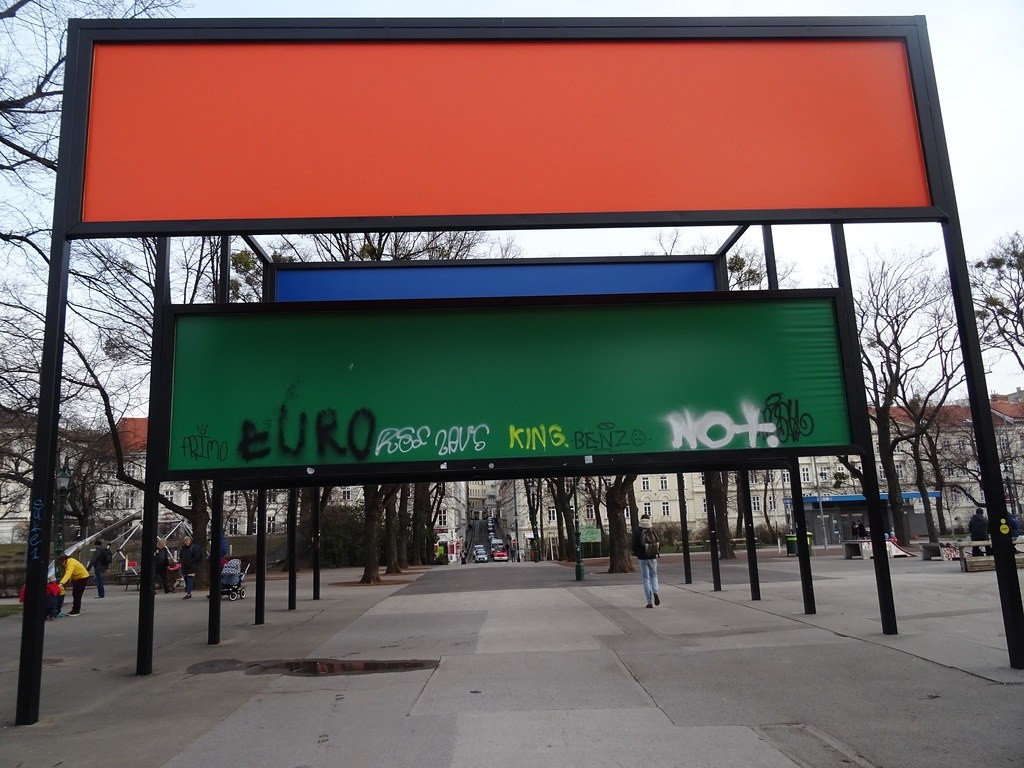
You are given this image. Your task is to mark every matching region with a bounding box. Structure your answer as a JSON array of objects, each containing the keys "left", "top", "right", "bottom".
[
  {"left": 487, "top": 516, "right": 509, "bottom": 561},
  {"left": 473, "top": 544, "right": 488, "bottom": 562}
]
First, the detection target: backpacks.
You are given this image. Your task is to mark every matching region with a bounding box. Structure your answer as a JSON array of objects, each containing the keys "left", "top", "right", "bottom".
[
  {"left": 640, "top": 528, "right": 659, "bottom": 559},
  {"left": 98, "top": 548, "right": 112, "bottom": 565}
]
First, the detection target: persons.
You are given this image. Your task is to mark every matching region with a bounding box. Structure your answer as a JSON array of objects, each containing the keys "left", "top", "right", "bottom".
[
  {"left": 461, "top": 541, "right": 515, "bottom": 565},
  {"left": 851, "top": 520, "right": 867, "bottom": 540},
  {"left": 631, "top": 514, "right": 661, "bottom": 608},
  {"left": 19, "top": 530, "right": 242, "bottom": 617},
  {"left": 969, "top": 507, "right": 1021, "bottom": 556}
]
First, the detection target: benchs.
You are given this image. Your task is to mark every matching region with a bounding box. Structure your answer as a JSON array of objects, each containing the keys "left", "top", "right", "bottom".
[
  {"left": 673, "top": 538, "right": 761, "bottom": 554},
  {"left": 953, "top": 539, "right": 1024, "bottom": 572},
  {"left": 116, "top": 574, "right": 140, "bottom": 591}
]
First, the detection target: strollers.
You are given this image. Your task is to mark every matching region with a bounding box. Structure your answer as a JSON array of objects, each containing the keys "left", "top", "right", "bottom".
[{"left": 221, "top": 555, "right": 254, "bottom": 601}]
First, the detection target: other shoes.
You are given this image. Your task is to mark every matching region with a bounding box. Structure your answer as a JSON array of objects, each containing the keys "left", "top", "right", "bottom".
[
  {"left": 647, "top": 604, "right": 653, "bottom": 608},
  {"left": 654, "top": 593, "right": 660, "bottom": 605},
  {"left": 182, "top": 595, "right": 191, "bottom": 599},
  {"left": 66, "top": 611, "right": 80, "bottom": 616},
  {"left": 165, "top": 588, "right": 173, "bottom": 594}
]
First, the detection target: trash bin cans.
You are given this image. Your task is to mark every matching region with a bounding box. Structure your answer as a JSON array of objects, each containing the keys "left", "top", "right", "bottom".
[
  {"left": 806, "top": 531, "right": 813, "bottom": 557},
  {"left": 786, "top": 534, "right": 799, "bottom": 556}
]
